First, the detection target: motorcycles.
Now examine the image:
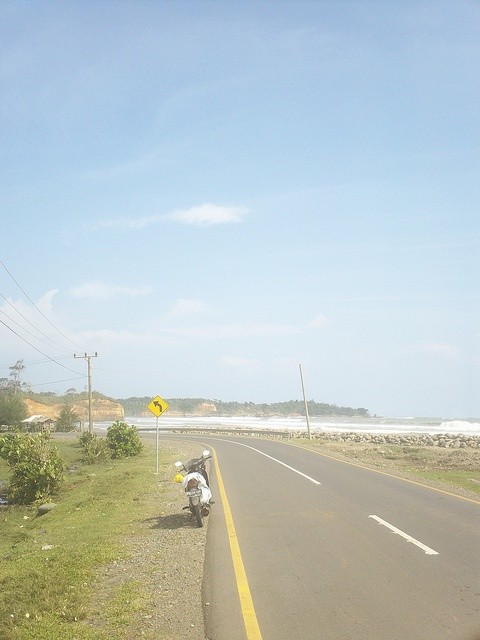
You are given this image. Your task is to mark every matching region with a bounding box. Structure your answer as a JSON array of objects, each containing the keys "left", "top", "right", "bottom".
[{"left": 174, "top": 450, "right": 215, "bottom": 527}]
